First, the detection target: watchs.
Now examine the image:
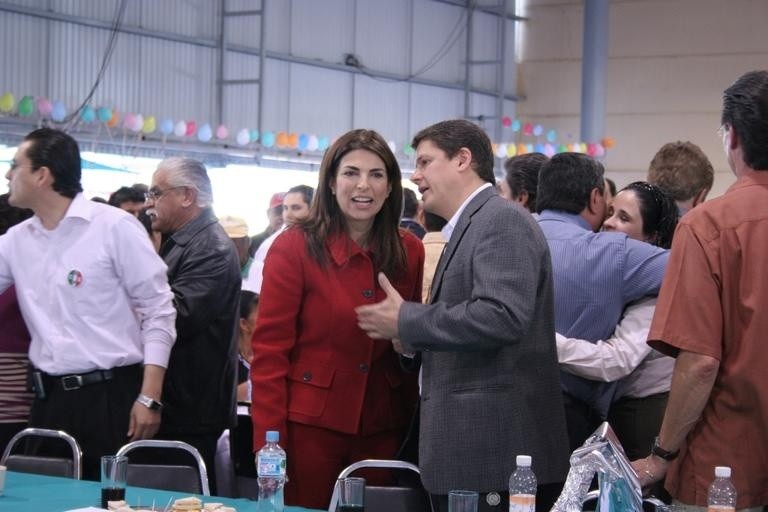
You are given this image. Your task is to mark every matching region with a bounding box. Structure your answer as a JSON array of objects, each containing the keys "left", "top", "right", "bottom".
[
  {"left": 650, "top": 436, "right": 681, "bottom": 460},
  {"left": 136, "top": 393, "right": 164, "bottom": 411}
]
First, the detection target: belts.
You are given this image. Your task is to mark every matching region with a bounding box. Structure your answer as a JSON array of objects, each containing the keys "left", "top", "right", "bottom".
[{"left": 47, "top": 370, "right": 115, "bottom": 392}]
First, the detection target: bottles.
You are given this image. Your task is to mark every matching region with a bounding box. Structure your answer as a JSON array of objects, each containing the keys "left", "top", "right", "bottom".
[
  {"left": 255, "top": 431, "right": 287, "bottom": 512},
  {"left": 707, "top": 466, "right": 737, "bottom": 512},
  {"left": 509, "top": 455, "right": 537, "bottom": 512}
]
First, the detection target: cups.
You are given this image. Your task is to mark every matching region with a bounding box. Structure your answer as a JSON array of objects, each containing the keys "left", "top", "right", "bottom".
[
  {"left": 448, "top": 490, "right": 478, "bottom": 512},
  {"left": 336, "top": 476, "right": 365, "bottom": 512},
  {"left": 0, "top": 465, "right": 6, "bottom": 497},
  {"left": 101, "top": 457, "right": 128, "bottom": 510}
]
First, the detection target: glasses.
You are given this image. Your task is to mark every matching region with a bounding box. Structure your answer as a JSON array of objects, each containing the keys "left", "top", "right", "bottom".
[
  {"left": 141, "top": 186, "right": 173, "bottom": 201},
  {"left": 8, "top": 159, "right": 34, "bottom": 171},
  {"left": 713, "top": 124, "right": 728, "bottom": 135},
  {"left": 633, "top": 180, "right": 666, "bottom": 221}
]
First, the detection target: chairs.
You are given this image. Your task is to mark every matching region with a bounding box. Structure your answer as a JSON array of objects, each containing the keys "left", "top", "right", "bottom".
[
  {"left": 109, "top": 438, "right": 209, "bottom": 496},
  {"left": 0, "top": 428, "right": 81, "bottom": 480},
  {"left": 328, "top": 459, "right": 434, "bottom": 512}
]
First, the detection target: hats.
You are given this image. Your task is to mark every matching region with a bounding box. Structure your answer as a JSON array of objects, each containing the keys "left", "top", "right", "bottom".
[
  {"left": 220, "top": 216, "right": 248, "bottom": 239},
  {"left": 268, "top": 192, "right": 286, "bottom": 210}
]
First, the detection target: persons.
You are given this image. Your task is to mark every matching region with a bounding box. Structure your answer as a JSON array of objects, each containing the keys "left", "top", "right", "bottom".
[
  {"left": 146, "top": 159, "right": 241, "bottom": 498},
  {"left": 92, "top": 179, "right": 156, "bottom": 239},
  {"left": 396, "top": 188, "right": 425, "bottom": 237},
  {"left": 0, "top": 130, "right": 176, "bottom": 481},
  {"left": 535, "top": 154, "right": 671, "bottom": 512},
  {"left": 229, "top": 286, "right": 261, "bottom": 435},
  {"left": 500, "top": 155, "right": 551, "bottom": 219},
  {"left": 647, "top": 144, "right": 715, "bottom": 219},
  {"left": 242, "top": 187, "right": 317, "bottom": 399},
  {"left": 241, "top": 194, "right": 286, "bottom": 329},
  {"left": 627, "top": 71, "right": 768, "bottom": 512},
  {"left": 251, "top": 131, "right": 423, "bottom": 512},
  {"left": 554, "top": 183, "right": 679, "bottom": 512},
  {"left": 355, "top": 121, "right": 570, "bottom": 512}
]
